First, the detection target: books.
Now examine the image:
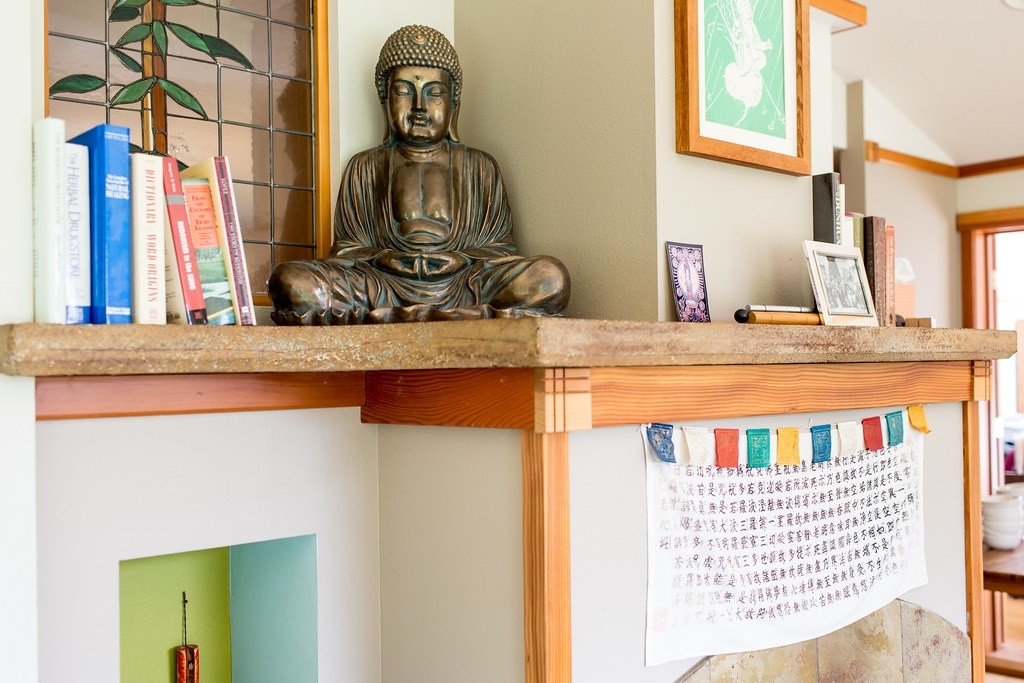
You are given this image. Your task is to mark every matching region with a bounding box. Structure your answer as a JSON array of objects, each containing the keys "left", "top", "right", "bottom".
[
  {"left": 32, "top": 118, "right": 257, "bottom": 325},
  {"left": 813, "top": 172, "right": 896, "bottom": 326}
]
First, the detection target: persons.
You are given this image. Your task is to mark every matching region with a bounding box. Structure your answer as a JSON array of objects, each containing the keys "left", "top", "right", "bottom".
[{"left": 268, "top": 25, "right": 570, "bottom": 324}]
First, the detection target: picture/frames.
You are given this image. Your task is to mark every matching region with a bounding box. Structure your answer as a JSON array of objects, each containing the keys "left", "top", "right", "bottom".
[
  {"left": 674, "top": 0, "right": 811, "bottom": 176},
  {"left": 666, "top": 240, "right": 719, "bottom": 322},
  {"left": 802, "top": 239, "right": 879, "bottom": 326}
]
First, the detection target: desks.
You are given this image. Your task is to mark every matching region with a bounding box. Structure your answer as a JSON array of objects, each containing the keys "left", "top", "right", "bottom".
[{"left": 982, "top": 537, "right": 1024, "bottom": 679}]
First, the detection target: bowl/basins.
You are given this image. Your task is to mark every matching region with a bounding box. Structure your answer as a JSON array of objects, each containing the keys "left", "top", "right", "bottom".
[{"left": 982, "top": 483, "right": 1024, "bottom": 549}]
